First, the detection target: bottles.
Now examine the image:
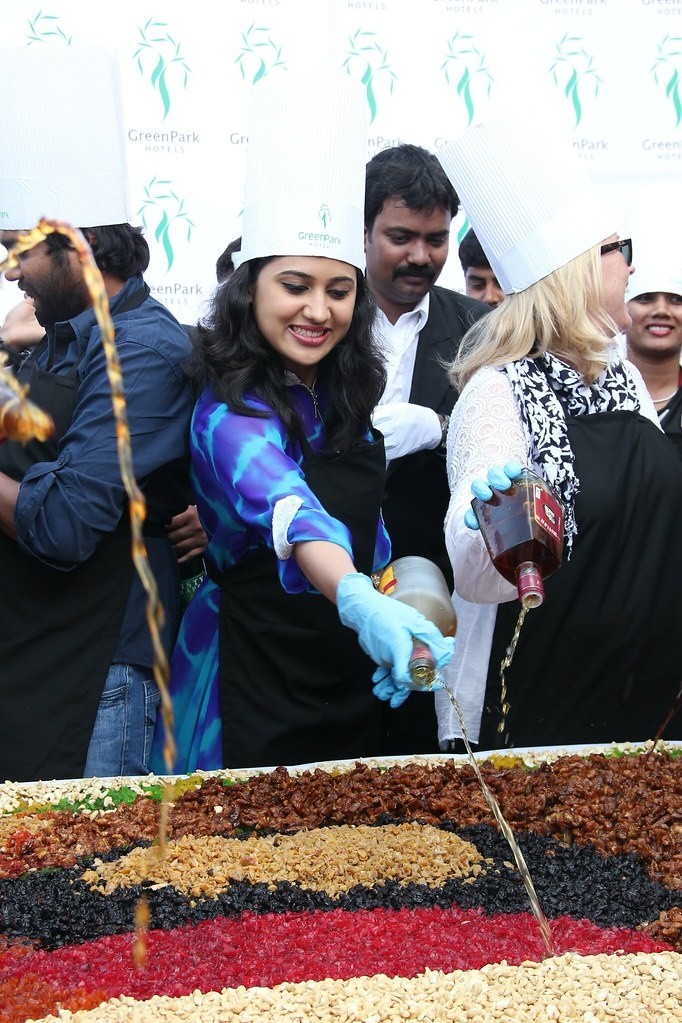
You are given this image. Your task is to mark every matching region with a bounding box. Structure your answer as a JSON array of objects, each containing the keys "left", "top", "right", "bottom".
[
  {"left": 471, "top": 469, "right": 564, "bottom": 608},
  {"left": 379, "top": 556, "right": 456, "bottom": 683}
]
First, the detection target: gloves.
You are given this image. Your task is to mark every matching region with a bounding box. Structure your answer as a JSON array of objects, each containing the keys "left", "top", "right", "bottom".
[
  {"left": 337, "top": 573, "right": 456, "bottom": 691},
  {"left": 465, "top": 459, "right": 520, "bottom": 531},
  {"left": 371, "top": 402, "right": 442, "bottom": 460},
  {"left": 372, "top": 663, "right": 412, "bottom": 708}
]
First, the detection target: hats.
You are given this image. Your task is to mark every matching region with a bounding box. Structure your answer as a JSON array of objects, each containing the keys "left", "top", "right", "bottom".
[
  {"left": 229, "top": 69, "right": 366, "bottom": 279},
  {"left": 611, "top": 154, "right": 682, "bottom": 301},
  {"left": 431, "top": 92, "right": 617, "bottom": 295},
  {"left": 0, "top": 24, "right": 130, "bottom": 230}
]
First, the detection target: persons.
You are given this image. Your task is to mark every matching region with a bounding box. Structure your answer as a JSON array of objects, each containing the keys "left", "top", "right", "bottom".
[{"left": 0, "top": 30, "right": 682, "bottom": 779}]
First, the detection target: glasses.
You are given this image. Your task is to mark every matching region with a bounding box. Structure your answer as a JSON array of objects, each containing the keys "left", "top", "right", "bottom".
[{"left": 601, "top": 238, "right": 633, "bottom": 267}]
